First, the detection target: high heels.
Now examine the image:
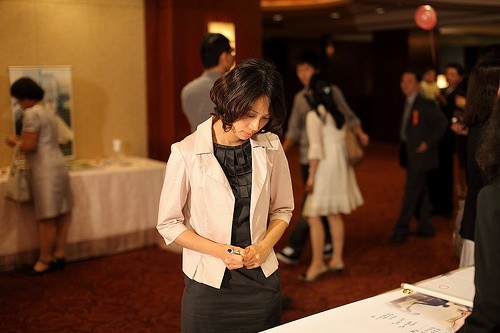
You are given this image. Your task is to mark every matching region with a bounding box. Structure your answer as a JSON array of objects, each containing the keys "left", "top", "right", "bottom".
[
  {"left": 298, "top": 264, "right": 329, "bottom": 282},
  {"left": 328, "top": 262, "right": 345, "bottom": 276}
]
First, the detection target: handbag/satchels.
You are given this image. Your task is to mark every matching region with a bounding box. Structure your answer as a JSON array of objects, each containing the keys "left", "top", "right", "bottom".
[
  {"left": 343, "top": 110, "right": 364, "bottom": 166},
  {"left": 4, "top": 141, "right": 34, "bottom": 203}
]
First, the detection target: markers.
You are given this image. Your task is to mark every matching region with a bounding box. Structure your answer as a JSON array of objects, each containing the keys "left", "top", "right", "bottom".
[{"left": 226, "top": 248, "right": 242, "bottom": 256}]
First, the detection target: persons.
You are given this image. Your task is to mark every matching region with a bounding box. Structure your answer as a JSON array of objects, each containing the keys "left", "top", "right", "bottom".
[
  {"left": 181, "top": 32, "right": 235, "bottom": 133},
  {"left": 5, "top": 77, "right": 74, "bottom": 275},
  {"left": 295, "top": 73, "right": 363, "bottom": 281},
  {"left": 14, "top": 72, "right": 71, "bottom": 156},
  {"left": 451, "top": 95, "right": 500, "bottom": 333},
  {"left": 387, "top": 66, "right": 448, "bottom": 243},
  {"left": 275, "top": 50, "right": 369, "bottom": 266},
  {"left": 418, "top": 60, "right": 500, "bottom": 269},
  {"left": 156, "top": 57, "right": 294, "bottom": 333}
]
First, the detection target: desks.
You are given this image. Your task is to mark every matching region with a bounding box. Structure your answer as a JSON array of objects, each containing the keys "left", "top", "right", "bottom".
[
  {"left": 0, "top": 155, "right": 166, "bottom": 272},
  {"left": 259, "top": 265, "right": 475, "bottom": 333}
]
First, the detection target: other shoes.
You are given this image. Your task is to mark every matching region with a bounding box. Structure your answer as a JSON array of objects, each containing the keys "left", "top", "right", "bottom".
[
  {"left": 275, "top": 246, "right": 300, "bottom": 265},
  {"left": 322, "top": 243, "right": 333, "bottom": 260},
  {"left": 409, "top": 229, "right": 436, "bottom": 239},
  {"left": 51, "top": 256, "right": 65, "bottom": 271},
  {"left": 22, "top": 259, "right": 58, "bottom": 276},
  {"left": 389, "top": 233, "right": 407, "bottom": 243}
]
